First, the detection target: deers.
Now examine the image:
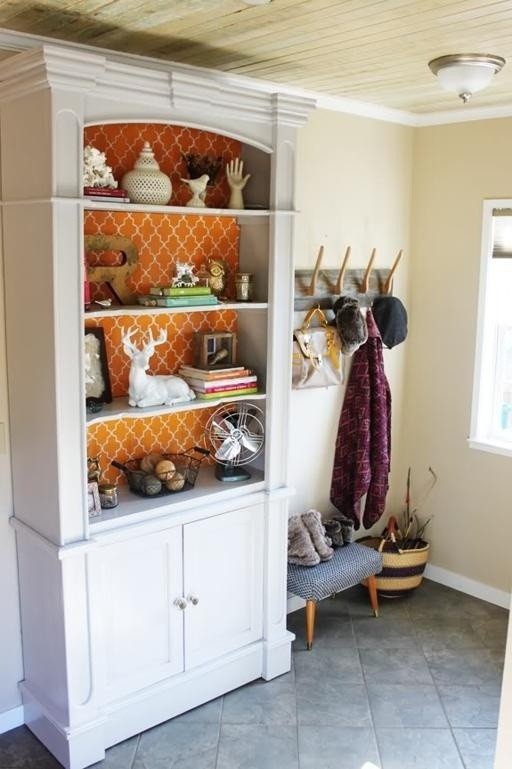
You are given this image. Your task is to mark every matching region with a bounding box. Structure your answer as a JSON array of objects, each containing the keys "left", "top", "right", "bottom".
[{"left": 119, "top": 322, "right": 197, "bottom": 407}]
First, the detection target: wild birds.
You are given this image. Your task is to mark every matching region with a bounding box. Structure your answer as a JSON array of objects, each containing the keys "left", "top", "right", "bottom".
[{"left": 179, "top": 174, "right": 211, "bottom": 198}]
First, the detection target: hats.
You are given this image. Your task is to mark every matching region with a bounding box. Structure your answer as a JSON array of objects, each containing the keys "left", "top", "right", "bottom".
[
  {"left": 333, "top": 295, "right": 369, "bottom": 360},
  {"left": 371, "top": 296, "right": 409, "bottom": 350}
]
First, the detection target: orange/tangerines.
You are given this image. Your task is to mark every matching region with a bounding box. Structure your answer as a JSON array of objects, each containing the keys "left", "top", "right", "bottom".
[{"left": 144, "top": 460, "right": 185, "bottom": 496}]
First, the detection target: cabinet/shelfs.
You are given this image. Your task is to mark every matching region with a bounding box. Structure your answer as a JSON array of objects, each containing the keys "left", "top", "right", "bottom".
[{"left": 0, "top": 44, "right": 318, "bottom": 769}]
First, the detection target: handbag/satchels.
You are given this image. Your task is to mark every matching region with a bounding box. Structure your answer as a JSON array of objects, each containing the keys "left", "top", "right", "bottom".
[{"left": 292, "top": 307, "right": 345, "bottom": 391}]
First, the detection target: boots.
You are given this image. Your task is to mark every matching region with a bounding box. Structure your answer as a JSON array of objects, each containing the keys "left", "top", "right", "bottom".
[{"left": 287, "top": 508, "right": 355, "bottom": 568}]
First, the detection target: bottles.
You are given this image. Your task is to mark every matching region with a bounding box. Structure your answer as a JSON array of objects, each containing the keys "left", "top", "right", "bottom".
[{"left": 97, "top": 484, "right": 120, "bottom": 509}]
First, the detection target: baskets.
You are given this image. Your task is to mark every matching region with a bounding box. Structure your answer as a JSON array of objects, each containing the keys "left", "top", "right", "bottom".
[
  {"left": 122, "top": 453, "right": 202, "bottom": 499},
  {"left": 354, "top": 536, "right": 430, "bottom": 600}
]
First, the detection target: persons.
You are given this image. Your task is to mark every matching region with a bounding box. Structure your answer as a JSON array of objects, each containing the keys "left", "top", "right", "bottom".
[{"left": 226, "top": 158, "right": 252, "bottom": 209}]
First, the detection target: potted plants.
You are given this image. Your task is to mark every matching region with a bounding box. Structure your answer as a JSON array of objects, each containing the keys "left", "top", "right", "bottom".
[{"left": 354, "top": 464, "right": 438, "bottom": 599}]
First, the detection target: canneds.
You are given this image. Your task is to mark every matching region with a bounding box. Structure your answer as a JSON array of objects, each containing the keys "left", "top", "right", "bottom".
[{"left": 98, "top": 484, "right": 118, "bottom": 509}]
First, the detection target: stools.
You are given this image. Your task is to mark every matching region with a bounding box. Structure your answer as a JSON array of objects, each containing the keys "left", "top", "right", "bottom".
[{"left": 287, "top": 541, "right": 383, "bottom": 651}]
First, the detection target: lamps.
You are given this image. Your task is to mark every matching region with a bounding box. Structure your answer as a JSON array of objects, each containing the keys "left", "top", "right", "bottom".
[{"left": 428, "top": 51, "right": 507, "bottom": 106}]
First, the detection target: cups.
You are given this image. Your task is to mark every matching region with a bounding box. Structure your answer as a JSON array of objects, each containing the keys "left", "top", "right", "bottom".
[{"left": 234, "top": 271, "right": 255, "bottom": 303}]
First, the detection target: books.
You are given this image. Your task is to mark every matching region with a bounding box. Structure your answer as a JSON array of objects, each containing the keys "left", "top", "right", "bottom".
[
  {"left": 84, "top": 194, "right": 131, "bottom": 203},
  {"left": 136, "top": 294, "right": 218, "bottom": 307},
  {"left": 177, "top": 361, "right": 259, "bottom": 399},
  {"left": 84, "top": 186, "right": 128, "bottom": 197},
  {"left": 148, "top": 286, "right": 212, "bottom": 296}
]
